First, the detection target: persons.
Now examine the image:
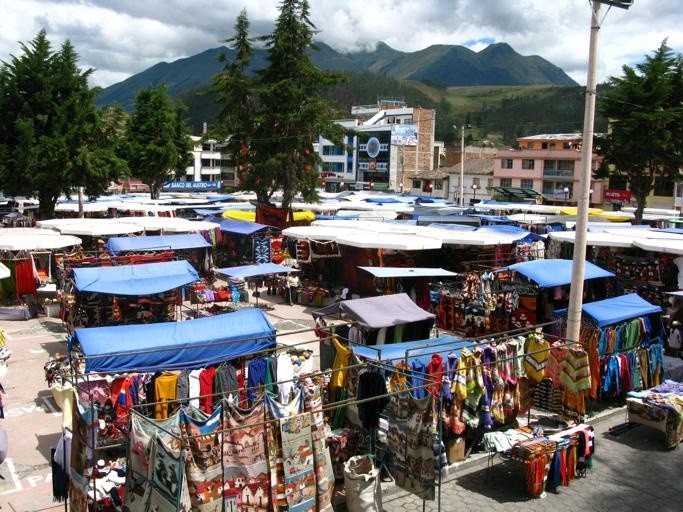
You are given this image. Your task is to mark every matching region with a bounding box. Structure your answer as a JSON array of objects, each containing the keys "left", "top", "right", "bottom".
[
  {"left": 286, "top": 271, "right": 303, "bottom": 303},
  {"left": 668, "top": 320, "right": 682, "bottom": 355}
]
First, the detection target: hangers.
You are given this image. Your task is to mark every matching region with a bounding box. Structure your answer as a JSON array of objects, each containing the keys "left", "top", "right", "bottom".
[
  {"left": 83, "top": 250, "right": 167, "bottom": 259},
  {"left": 104, "top": 361, "right": 231, "bottom": 379},
  {"left": 610, "top": 342, "right": 643, "bottom": 358},
  {"left": 614, "top": 322, "right": 620, "bottom": 331},
  {"left": 117, "top": 385, "right": 278, "bottom": 423}
]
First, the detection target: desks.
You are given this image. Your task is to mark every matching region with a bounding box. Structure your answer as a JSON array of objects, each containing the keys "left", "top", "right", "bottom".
[
  {"left": 0, "top": 304, "right": 34, "bottom": 320},
  {"left": 626, "top": 379, "right": 683, "bottom": 449},
  {"left": 486, "top": 432, "right": 586, "bottom": 495}
]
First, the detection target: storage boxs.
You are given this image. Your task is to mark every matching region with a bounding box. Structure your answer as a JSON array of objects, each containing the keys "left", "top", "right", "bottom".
[{"left": 447, "top": 437, "right": 465, "bottom": 465}]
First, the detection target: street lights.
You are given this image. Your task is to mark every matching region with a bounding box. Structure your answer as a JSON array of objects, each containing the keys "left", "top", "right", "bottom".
[{"left": 337, "top": 181, "right": 595, "bottom": 207}]
